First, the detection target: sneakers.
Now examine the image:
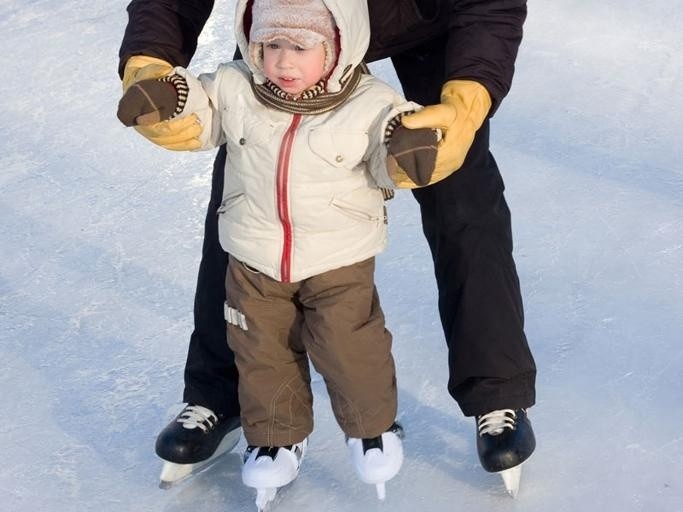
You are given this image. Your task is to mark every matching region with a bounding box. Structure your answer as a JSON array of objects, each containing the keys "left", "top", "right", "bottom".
[
  {"left": 155, "top": 404, "right": 241, "bottom": 464},
  {"left": 241, "top": 438, "right": 307, "bottom": 489},
  {"left": 348, "top": 420, "right": 404, "bottom": 481},
  {"left": 477, "top": 408, "right": 536, "bottom": 473}
]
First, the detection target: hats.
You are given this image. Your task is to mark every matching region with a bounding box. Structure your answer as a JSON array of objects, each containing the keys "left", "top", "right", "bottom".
[{"left": 247, "top": 1, "right": 339, "bottom": 84}]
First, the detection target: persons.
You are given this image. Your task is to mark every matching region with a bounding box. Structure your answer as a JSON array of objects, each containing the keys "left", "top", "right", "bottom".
[
  {"left": 117, "top": 0, "right": 537, "bottom": 474},
  {"left": 117, "top": 0, "right": 442, "bottom": 488}
]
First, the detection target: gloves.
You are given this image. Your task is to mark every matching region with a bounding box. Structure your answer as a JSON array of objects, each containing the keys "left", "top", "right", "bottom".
[
  {"left": 122, "top": 54, "right": 202, "bottom": 151},
  {"left": 390, "top": 126, "right": 438, "bottom": 186},
  {"left": 391, "top": 80, "right": 492, "bottom": 189},
  {"left": 117, "top": 78, "right": 178, "bottom": 127}
]
